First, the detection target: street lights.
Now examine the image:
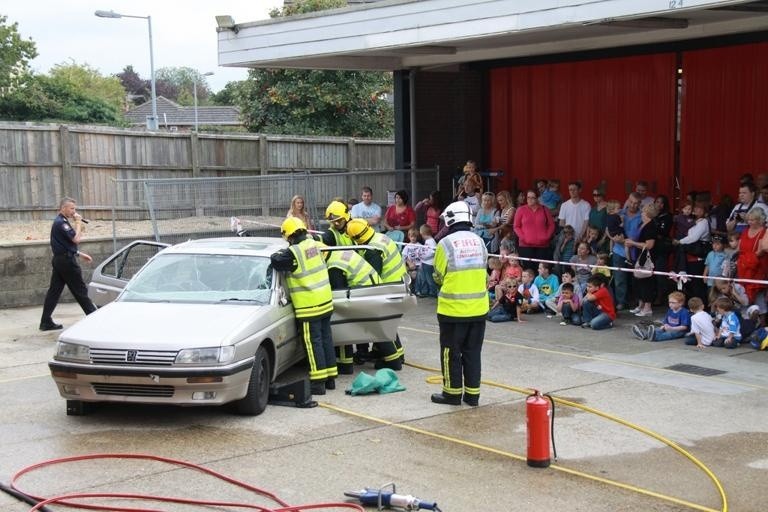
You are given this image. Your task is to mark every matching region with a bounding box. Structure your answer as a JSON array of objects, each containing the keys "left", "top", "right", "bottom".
[
  {"left": 94, "top": 10, "right": 158, "bottom": 130},
  {"left": 192, "top": 72, "right": 215, "bottom": 131}
]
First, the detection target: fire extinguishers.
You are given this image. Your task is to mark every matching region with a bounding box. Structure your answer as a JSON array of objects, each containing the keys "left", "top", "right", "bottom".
[{"left": 526, "top": 387, "right": 557, "bottom": 468}]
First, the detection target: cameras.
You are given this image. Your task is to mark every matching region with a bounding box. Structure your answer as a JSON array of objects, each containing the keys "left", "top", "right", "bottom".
[{"left": 736, "top": 214, "right": 745, "bottom": 223}]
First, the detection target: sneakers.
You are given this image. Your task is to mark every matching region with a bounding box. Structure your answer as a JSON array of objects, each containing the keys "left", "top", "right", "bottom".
[
  {"left": 326, "top": 378, "right": 335, "bottom": 390},
  {"left": 431, "top": 394, "right": 461, "bottom": 405},
  {"left": 311, "top": 381, "right": 326, "bottom": 395},
  {"left": 559, "top": 319, "right": 570, "bottom": 325},
  {"left": 463, "top": 394, "right": 478, "bottom": 406},
  {"left": 647, "top": 325, "right": 655, "bottom": 341},
  {"left": 632, "top": 325, "right": 645, "bottom": 341},
  {"left": 608, "top": 251, "right": 613, "bottom": 258},
  {"left": 375, "top": 360, "right": 401, "bottom": 370},
  {"left": 624, "top": 259, "right": 633, "bottom": 265},
  {"left": 353, "top": 352, "right": 368, "bottom": 364},
  {"left": 337, "top": 363, "right": 353, "bottom": 374}
]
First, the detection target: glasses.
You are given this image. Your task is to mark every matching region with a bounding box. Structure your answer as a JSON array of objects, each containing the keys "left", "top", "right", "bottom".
[
  {"left": 484, "top": 193, "right": 494, "bottom": 196},
  {"left": 508, "top": 285, "right": 517, "bottom": 288},
  {"left": 593, "top": 194, "right": 600, "bottom": 197}
]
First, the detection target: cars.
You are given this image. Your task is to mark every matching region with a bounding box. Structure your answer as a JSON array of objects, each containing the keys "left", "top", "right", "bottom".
[{"left": 49, "top": 236, "right": 419, "bottom": 415}]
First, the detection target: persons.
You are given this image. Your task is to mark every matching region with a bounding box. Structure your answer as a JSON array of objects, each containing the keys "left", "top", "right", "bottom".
[
  {"left": 269, "top": 216, "right": 338, "bottom": 396},
  {"left": 322, "top": 200, "right": 412, "bottom": 371},
  {"left": 428, "top": 200, "right": 490, "bottom": 408},
  {"left": 288, "top": 186, "right": 451, "bottom": 298},
  {"left": 39, "top": 195, "right": 97, "bottom": 332},
  {"left": 457, "top": 159, "right": 767, "bottom": 350}
]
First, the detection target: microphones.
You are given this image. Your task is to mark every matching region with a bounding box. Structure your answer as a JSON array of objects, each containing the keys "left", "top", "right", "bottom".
[{"left": 81, "top": 219, "right": 88, "bottom": 224}]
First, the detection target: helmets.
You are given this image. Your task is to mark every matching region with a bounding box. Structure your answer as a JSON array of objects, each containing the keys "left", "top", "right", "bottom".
[
  {"left": 280, "top": 216, "right": 309, "bottom": 240},
  {"left": 347, "top": 218, "right": 375, "bottom": 244},
  {"left": 439, "top": 201, "right": 474, "bottom": 227},
  {"left": 326, "top": 201, "right": 350, "bottom": 227}
]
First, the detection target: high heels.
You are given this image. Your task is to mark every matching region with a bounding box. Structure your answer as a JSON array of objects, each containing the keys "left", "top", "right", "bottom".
[
  {"left": 635, "top": 310, "right": 652, "bottom": 317},
  {"left": 629, "top": 307, "right": 642, "bottom": 313}
]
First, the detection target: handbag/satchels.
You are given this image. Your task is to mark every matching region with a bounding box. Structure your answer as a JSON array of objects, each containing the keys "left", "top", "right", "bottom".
[
  {"left": 634, "top": 259, "right": 654, "bottom": 278},
  {"left": 687, "top": 240, "right": 712, "bottom": 258}
]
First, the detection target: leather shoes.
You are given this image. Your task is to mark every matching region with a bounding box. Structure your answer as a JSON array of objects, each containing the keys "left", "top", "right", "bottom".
[{"left": 39, "top": 322, "right": 62, "bottom": 332}]
